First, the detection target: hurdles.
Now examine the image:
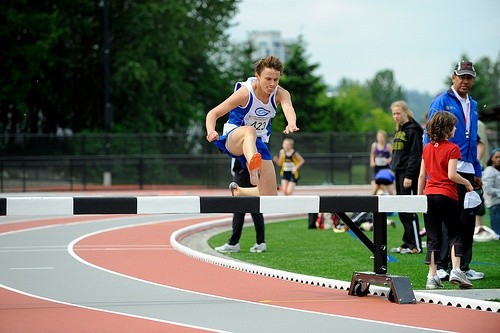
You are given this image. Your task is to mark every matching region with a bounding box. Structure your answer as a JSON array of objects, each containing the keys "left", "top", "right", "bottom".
[{"left": 0, "top": 195, "right": 428, "bottom": 304}]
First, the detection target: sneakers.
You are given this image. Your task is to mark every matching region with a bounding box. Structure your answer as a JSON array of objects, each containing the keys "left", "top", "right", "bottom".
[
  {"left": 449, "top": 269, "right": 473, "bottom": 289},
  {"left": 215, "top": 243, "right": 240, "bottom": 252},
  {"left": 462, "top": 270, "right": 484, "bottom": 280},
  {"left": 229, "top": 182, "right": 238, "bottom": 196},
  {"left": 436, "top": 269, "right": 449, "bottom": 280},
  {"left": 249, "top": 153, "right": 261, "bottom": 185},
  {"left": 250, "top": 243, "right": 266, "bottom": 252},
  {"left": 426, "top": 276, "right": 444, "bottom": 289},
  {"left": 401, "top": 248, "right": 418, "bottom": 254},
  {"left": 390, "top": 246, "right": 401, "bottom": 252}
]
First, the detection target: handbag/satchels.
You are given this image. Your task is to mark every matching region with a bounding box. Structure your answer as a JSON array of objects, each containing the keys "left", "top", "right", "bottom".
[
  {"left": 308, "top": 213, "right": 318, "bottom": 228},
  {"left": 316, "top": 213, "right": 339, "bottom": 229},
  {"left": 464, "top": 191, "right": 482, "bottom": 209}
]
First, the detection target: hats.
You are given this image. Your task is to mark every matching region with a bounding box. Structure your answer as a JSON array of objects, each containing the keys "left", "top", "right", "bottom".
[{"left": 454, "top": 60, "right": 476, "bottom": 77}]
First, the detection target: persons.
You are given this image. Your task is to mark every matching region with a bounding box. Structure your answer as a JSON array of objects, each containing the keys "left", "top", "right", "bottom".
[
  {"left": 277, "top": 138, "right": 305, "bottom": 196},
  {"left": 370, "top": 101, "right": 500, "bottom": 254},
  {"left": 416, "top": 112, "right": 474, "bottom": 289},
  {"left": 206, "top": 56, "right": 300, "bottom": 186},
  {"left": 423, "top": 62, "right": 485, "bottom": 280},
  {"left": 215, "top": 157, "right": 267, "bottom": 252}
]
